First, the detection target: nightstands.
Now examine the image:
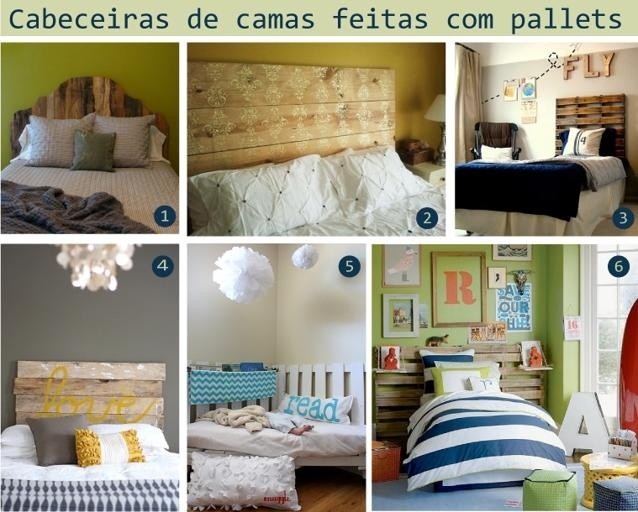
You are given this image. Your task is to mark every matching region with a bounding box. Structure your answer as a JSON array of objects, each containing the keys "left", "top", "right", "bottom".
[{"left": 403, "top": 160, "right": 445, "bottom": 194}]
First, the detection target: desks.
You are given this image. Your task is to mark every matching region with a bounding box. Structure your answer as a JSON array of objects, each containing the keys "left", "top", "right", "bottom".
[{"left": 579, "top": 451, "right": 638, "bottom": 509}]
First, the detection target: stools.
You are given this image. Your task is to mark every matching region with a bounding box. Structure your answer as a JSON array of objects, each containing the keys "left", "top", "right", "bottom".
[
  {"left": 591, "top": 476, "right": 638, "bottom": 512},
  {"left": 522, "top": 469, "right": 578, "bottom": 512}
]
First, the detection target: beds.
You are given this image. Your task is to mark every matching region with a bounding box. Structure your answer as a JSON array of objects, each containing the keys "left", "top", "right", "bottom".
[
  {"left": 403, "top": 390, "right": 566, "bottom": 493},
  {"left": 187, "top": 359, "right": 369, "bottom": 480},
  {"left": 1, "top": 75, "right": 178, "bottom": 234},
  {"left": 2, "top": 358, "right": 180, "bottom": 511},
  {"left": 188, "top": 61, "right": 445, "bottom": 237},
  {"left": 456, "top": 95, "right": 628, "bottom": 236}
]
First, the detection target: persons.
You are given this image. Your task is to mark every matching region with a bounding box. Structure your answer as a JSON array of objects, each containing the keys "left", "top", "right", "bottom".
[
  {"left": 384, "top": 348, "right": 397, "bottom": 371},
  {"left": 527, "top": 345, "right": 542, "bottom": 368},
  {"left": 265, "top": 412, "right": 313, "bottom": 436},
  {"left": 471, "top": 320, "right": 505, "bottom": 343}
]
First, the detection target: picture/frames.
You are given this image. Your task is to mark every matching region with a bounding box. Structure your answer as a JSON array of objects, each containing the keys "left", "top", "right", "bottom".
[
  {"left": 429, "top": 249, "right": 487, "bottom": 328},
  {"left": 380, "top": 245, "right": 424, "bottom": 289},
  {"left": 520, "top": 77, "right": 536, "bottom": 99},
  {"left": 380, "top": 292, "right": 422, "bottom": 339},
  {"left": 491, "top": 245, "right": 534, "bottom": 263},
  {"left": 488, "top": 266, "right": 508, "bottom": 290},
  {"left": 503, "top": 79, "right": 519, "bottom": 101}
]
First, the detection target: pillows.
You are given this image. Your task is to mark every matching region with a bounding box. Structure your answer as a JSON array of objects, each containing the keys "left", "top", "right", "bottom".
[
  {"left": 480, "top": 144, "right": 513, "bottom": 162},
  {"left": 417, "top": 347, "right": 501, "bottom": 398},
  {"left": 559, "top": 126, "right": 616, "bottom": 158},
  {"left": 280, "top": 392, "right": 354, "bottom": 424},
  {"left": 23, "top": 114, "right": 158, "bottom": 173},
  {"left": 188, "top": 145, "right": 419, "bottom": 236},
  {"left": 186, "top": 452, "right": 301, "bottom": 512},
  {"left": 1, "top": 415, "right": 168, "bottom": 469}
]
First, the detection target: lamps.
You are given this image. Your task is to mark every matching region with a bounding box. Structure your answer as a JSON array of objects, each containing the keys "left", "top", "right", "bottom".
[
  {"left": 54, "top": 244, "right": 144, "bottom": 295},
  {"left": 425, "top": 94, "right": 445, "bottom": 166}
]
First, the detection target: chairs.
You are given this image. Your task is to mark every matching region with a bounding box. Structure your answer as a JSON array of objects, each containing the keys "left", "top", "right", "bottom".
[{"left": 470, "top": 121, "right": 521, "bottom": 161}]
